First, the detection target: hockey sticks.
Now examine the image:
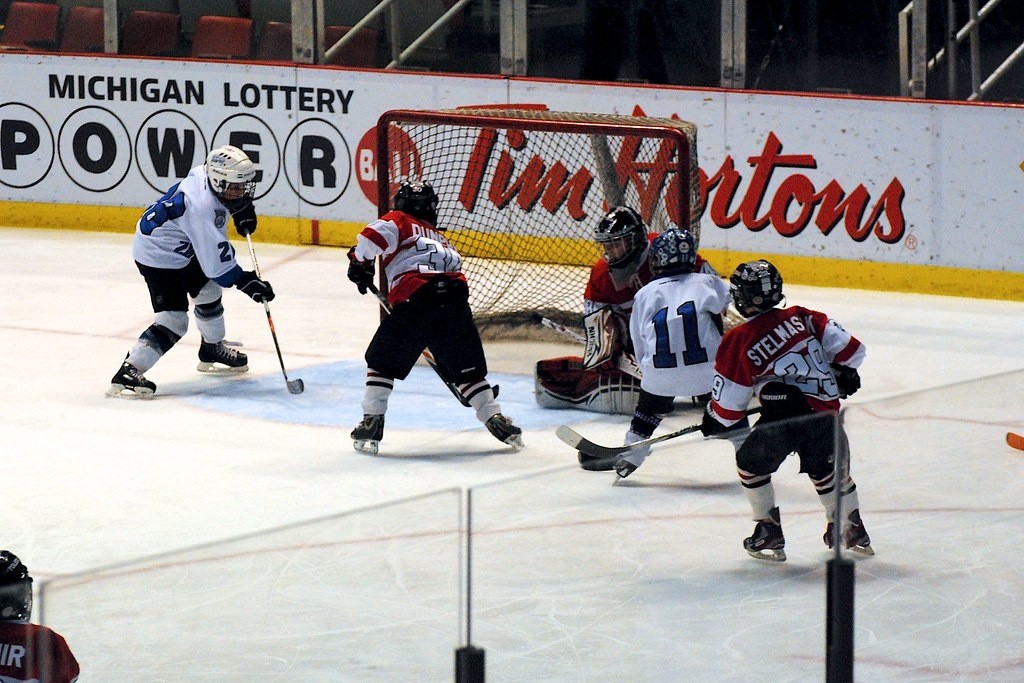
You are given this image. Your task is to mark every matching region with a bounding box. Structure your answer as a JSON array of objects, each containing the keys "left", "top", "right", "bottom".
[
  {"left": 1006, "top": 432, "right": 1024, "bottom": 450},
  {"left": 243, "top": 226, "right": 305, "bottom": 394},
  {"left": 369, "top": 282, "right": 499, "bottom": 408},
  {"left": 555, "top": 406, "right": 761, "bottom": 458},
  {"left": 529, "top": 311, "right": 642, "bottom": 379}
]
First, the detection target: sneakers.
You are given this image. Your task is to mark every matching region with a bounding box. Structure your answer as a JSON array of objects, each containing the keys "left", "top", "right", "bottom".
[
  {"left": 485, "top": 413, "right": 523, "bottom": 452},
  {"left": 105, "top": 352, "right": 156, "bottom": 400},
  {"left": 350, "top": 414, "right": 384, "bottom": 454},
  {"left": 196, "top": 335, "right": 249, "bottom": 373},
  {"left": 743, "top": 506, "right": 786, "bottom": 561},
  {"left": 612, "top": 431, "right": 653, "bottom": 487},
  {"left": 823, "top": 508, "right": 875, "bottom": 556}
]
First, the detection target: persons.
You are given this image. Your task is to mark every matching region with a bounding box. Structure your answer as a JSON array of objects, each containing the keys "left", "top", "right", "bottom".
[
  {"left": 346, "top": 180, "right": 524, "bottom": 457},
  {"left": 535, "top": 204, "right": 715, "bottom": 415},
  {"left": 704, "top": 260, "right": 875, "bottom": 562},
  {"left": 612, "top": 228, "right": 751, "bottom": 487},
  {"left": 105, "top": 145, "right": 275, "bottom": 401},
  {"left": 0, "top": 548, "right": 80, "bottom": 683}
]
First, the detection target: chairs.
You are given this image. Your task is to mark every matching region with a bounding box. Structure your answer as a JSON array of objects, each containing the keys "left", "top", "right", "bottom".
[
  {"left": 382, "top": 0, "right": 452, "bottom": 68},
  {"left": 256, "top": 22, "right": 293, "bottom": 62},
  {"left": 325, "top": 26, "right": 386, "bottom": 68},
  {"left": 0, "top": 2, "right": 62, "bottom": 50},
  {"left": 58, "top": 6, "right": 105, "bottom": 53},
  {"left": 56, "top": 0, "right": 382, "bottom": 33},
  {"left": 120, "top": 10, "right": 182, "bottom": 56},
  {"left": 189, "top": 15, "right": 255, "bottom": 60}
]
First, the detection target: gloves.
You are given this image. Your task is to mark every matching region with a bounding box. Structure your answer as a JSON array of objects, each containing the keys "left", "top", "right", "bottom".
[
  {"left": 229, "top": 192, "right": 257, "bottom": 237},
  {"left": 828, "top": 361, "right": 861, "bottom": 400},
  {"left": 345, "top": 245, "right": 376, "bottom": 296},
  {"left": 701, "top": 399, "right": 731, "bottom": 439},
  {"left": 236, "top": 270, "right": 275, "bottom": 304}
]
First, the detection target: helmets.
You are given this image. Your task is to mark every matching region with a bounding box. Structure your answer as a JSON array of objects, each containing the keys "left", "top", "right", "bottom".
[
  {"left": 206, "top": 145, "right": 257, "bottom": 214},
  {"left": 728, "top": 259, "right": 783, "bottom": 318},
  {"left": 393, "top": 180, "right": 439, "bottom": 228},
  {"left": 0, "top": 549, "right": 33, "bottom": 622},
  {"left": 647, "top": 227, "right": 698, "bottom": 275},
  {"left": 592, "top": 205, "right": 648, "bottom": 273}
]
